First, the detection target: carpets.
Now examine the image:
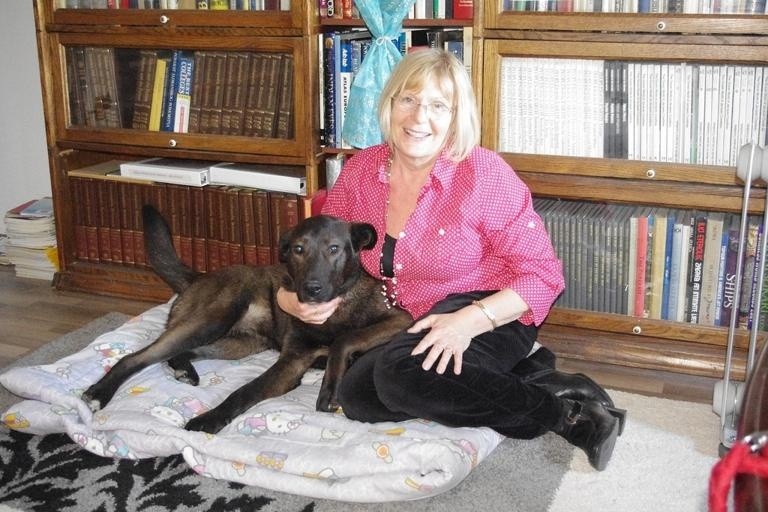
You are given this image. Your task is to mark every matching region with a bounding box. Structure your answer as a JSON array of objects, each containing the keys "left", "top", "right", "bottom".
[{"left": 0, "top": 311, "right": 736, "bottom": 512}]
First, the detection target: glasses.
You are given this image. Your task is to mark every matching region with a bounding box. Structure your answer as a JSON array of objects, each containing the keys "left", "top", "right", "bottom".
[{"left": 391, "top": 93, "right": 453, "bottom": 120}]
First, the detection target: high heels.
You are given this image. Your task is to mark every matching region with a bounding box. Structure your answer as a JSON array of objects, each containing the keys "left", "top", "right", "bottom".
[
  {"left": 551, "top": 398, "right": 627, "bottom": 471},
  {"left": 511, "top": 347, "right": 613, "bottom": 406}
]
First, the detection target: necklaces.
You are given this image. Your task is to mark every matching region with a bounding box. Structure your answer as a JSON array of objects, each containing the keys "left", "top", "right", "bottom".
[{"left": 382, "top": 159, "right": 439, "bottom": 310}]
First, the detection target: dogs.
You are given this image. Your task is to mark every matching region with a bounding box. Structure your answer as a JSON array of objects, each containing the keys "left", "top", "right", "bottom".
[{"left": 81, "top": 204, "right": 414, "bottom": 435}]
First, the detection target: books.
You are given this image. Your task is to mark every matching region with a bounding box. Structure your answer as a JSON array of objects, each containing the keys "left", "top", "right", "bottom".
[
  {"left": 503, "top": 0, "right": 767, "bottom": 35},
  {"left": 1, "top": 195, "right": 59, "bottom": 280},
  {"left": 53, "top": 0, "right": 290, "bottom": 12},
  {"left": 320, "top": 0, "right": 473, "bottom": 20},
  {"left": 316, "top": 28, "right": 472, "bottom": 151},
  {"left": 66, "top": 157, "right": 306, "bottom": 274},
  {"left": 66, "top": 48, "right": 294, "bottom": 140},
  {"left": 305, "top": 154, "right": 352, "bottom": 220},
  {"left": 498, "top": 56, "right": 767, "bottom": 167},
  {"left": 533, "top": 197, "right": 767, "bottom": 333}
]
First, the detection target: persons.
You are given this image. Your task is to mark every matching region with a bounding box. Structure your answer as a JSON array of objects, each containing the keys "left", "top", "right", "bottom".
[{"left": 275, "top": 47, "right": 629, "bottom": 473}]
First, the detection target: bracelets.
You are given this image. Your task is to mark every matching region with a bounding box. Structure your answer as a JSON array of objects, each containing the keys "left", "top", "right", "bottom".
[{"left": 471, "top": 299, "right": 496, "bottom": 329}]
